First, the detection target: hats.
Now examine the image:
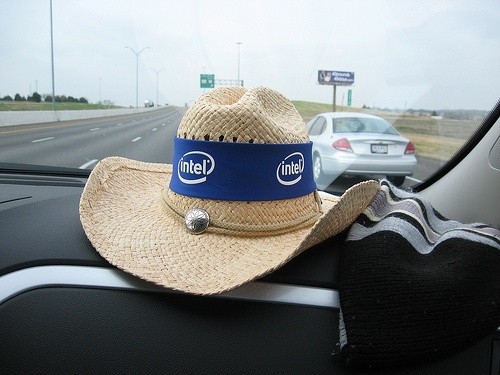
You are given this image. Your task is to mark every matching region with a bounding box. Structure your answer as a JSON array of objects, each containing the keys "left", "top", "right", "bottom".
[{"left": 78, "top": 85, "right": 381, "bottom": 295}]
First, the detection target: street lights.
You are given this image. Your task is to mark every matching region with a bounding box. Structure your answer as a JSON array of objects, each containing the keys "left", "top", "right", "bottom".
[
  {"left": 150, "top": 68, "right": 166, "bottom": 107},
  {"left": 236, "top": 42, "right": 243, "bottom": 85},
  {"left": 125, "top": 46, "right": 152, "bottom": 109}
]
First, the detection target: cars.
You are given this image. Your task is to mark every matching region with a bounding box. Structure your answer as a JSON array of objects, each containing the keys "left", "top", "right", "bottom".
[{"left": 305, "top": 112, "right": 418, "bottom": 188}]
